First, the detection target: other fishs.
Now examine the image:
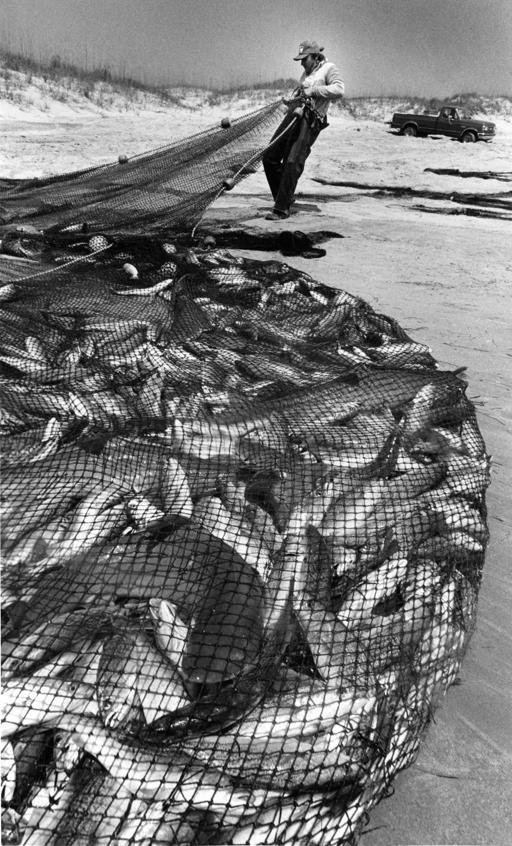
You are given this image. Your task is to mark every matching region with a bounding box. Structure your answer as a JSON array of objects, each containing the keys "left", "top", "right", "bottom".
[{"left": 4, "top": 217, "right": 487, "bottom": 846}]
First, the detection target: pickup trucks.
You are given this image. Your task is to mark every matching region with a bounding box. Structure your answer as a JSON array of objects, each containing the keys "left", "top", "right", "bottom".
[{"left": 391, "top": 106, "right": 497, "bottom": 142}]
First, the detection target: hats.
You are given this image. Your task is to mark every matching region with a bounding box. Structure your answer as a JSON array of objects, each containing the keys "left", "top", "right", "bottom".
[{"left": 293, "top": 40, "right": 325, "bottom": 60}]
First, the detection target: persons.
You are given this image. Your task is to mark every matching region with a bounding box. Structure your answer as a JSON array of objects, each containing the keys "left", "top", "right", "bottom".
[{"left": 260, "top": 38, "right": 346, "bottom": 221}]
